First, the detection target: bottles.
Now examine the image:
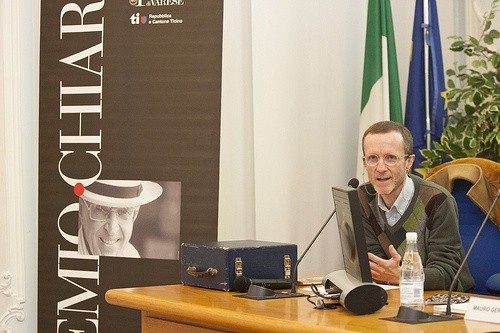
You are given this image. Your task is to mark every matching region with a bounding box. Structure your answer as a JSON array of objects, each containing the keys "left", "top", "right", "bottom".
[{"left": 399, "top": 232, "right": 425, "bottom": 311}]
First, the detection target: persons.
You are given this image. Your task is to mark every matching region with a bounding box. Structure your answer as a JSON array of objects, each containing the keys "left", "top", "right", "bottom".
[
  {"left": 354, "top": 121, "right": 478, "bottom": 295},
  {"left": 77, "top": 180, "right": 163, "bottom": 258}
]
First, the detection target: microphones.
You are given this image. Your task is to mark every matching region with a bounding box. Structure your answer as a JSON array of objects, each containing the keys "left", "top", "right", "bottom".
[
  {"left": 380, "top": 188, "right": 500, "bottom": 324},
  {"left": 234, "top": 276, "right": 292, "bottom": 293},
  {"left": 232, "top": 178, "right": 359, "bottom": 300}
]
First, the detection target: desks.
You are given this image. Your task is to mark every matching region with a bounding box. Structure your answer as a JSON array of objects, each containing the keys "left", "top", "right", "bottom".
[{"left": 104, "top": 274, "right": 500, "bottom": 333}]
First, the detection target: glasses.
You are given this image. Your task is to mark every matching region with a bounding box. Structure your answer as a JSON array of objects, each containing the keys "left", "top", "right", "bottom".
[
  {"left": 82, "top": 199, "right": 139, "bottom": 222},
  {"left": 361, "top": 153, "right": 412, "bottom": 166}
]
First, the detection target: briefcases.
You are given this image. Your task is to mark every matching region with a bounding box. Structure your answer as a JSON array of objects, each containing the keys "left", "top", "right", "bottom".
[{"left": 180, "top": 239, "right": 297, "bottom": 292}]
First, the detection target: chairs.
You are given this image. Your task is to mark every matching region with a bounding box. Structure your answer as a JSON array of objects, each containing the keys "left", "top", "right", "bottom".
[{"left": 423, "top": 157, "right": 500, "bottom": 298}]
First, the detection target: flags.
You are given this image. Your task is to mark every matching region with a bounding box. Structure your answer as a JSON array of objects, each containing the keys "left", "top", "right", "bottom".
[
  {"left": 405, "top": 0, "right": 449, "bottom": 179},
  {"left": 356, "top": 0, "right": 403, "bottom": 189}
]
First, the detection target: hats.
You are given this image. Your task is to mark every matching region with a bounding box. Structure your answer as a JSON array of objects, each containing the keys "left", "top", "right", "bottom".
[{"left": 80, "top": 180, "right": 164, "bottom": 207}]
